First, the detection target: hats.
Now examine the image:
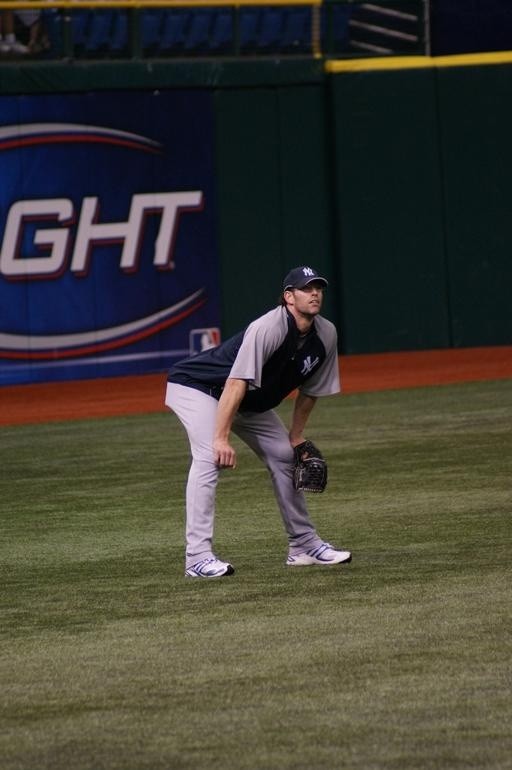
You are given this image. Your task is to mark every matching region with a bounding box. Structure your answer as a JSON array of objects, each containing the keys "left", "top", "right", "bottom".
[{"left": 282, "top": 266, "right": 329, "bottom": 291}]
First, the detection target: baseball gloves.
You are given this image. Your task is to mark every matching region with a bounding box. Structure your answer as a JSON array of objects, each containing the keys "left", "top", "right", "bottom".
[{"left": 294, "top": 439, "right": 328, "bottom": 493}]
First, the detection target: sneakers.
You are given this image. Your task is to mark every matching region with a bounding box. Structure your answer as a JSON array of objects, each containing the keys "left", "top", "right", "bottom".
[
  {"left": 184, "top": 558, "right": 234, "bottom": 578},
  {"left": 286, "top": 543, "right": 352, "bottom": 566}
]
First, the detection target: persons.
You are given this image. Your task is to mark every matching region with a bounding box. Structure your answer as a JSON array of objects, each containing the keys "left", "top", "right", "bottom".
[
  {"left": 164, "top": 266, "right": 352, "bottom": 579},
  {"left": 0, "top": 9, "right": 51, "bottom": 55}
]
